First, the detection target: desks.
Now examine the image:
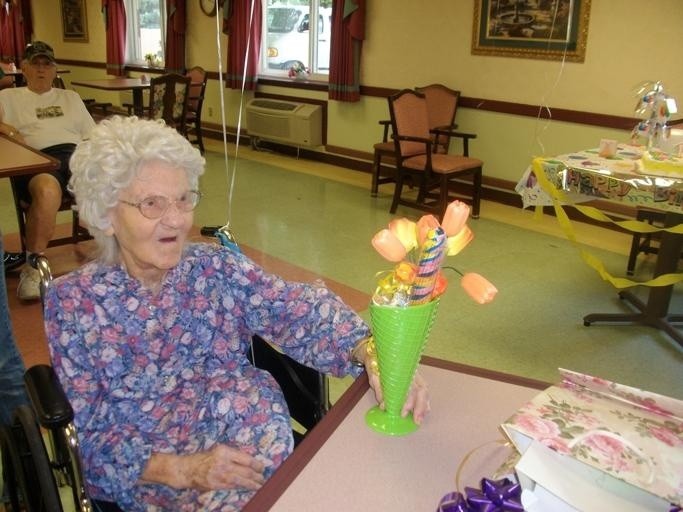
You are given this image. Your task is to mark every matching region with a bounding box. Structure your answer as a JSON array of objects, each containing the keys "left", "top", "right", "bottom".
[
  {"left": 0, "top": 64, "right": 71, "bottom": 87},
  {"left": 0, "top": 133, "right": 61, "bottom": 271},
  {"left": 71, "top": 77, "right": 151, "bottom": 118},
  {"left": 239, "top": 353, "right": 682, "bottom": 512},
  {"left": 516, "top": 138, "right": 683, "bottom": 348}
]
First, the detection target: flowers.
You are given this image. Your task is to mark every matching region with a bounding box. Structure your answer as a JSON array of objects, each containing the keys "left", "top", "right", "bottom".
[{"left": 370, "top": 198, "right": 500, "bottom": 308}]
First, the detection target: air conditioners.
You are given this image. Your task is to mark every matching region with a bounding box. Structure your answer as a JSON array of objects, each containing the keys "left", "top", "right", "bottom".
[{"left": 244, "top": 97, "right": 323, "bottom": 148}]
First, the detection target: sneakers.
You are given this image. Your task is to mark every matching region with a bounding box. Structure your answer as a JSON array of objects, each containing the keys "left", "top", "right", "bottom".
[{"left": 17, "top": 265, "right": 44, "bottom": 302}]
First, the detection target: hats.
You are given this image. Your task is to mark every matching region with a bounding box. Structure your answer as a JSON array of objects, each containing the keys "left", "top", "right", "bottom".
[{"left": 23, "top": 41, "right": 57, "bottom": 65}]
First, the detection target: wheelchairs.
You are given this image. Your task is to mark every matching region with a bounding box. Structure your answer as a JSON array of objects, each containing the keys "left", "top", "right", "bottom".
[{"left": 0, "top": 225, "right": 334, "bottom": 512}]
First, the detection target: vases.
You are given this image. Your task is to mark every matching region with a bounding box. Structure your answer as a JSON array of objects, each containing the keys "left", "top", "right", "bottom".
[{"left": 366, "top": 297, "right": 439, "bottom": 435}]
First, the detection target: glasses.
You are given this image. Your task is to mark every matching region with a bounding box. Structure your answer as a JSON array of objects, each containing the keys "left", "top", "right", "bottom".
[{"left": 118, "top": 191, "right": 200, "bottom": 219}]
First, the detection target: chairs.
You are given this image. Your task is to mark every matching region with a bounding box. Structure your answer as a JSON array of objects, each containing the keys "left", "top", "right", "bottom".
[
  {"left": 386, "top": 88, "right": 486, "bottom": 226},
  {"left": 7, "top": 176, "right": 94, "bottom": 257},
  {"left": 178, "top": 66, "right": 213, "bottom": 153},
  {"left": 625, "top": 118, "right": 683, "bottom": 277},
  {"left": 122, "top": 72, "right": 188, "bottom": 137},
  {"left": 51, "top": 77, "right": 113, "bottom": 118},
  {"left": 371, "top": 83, "right": 461, "bottom": 197}
]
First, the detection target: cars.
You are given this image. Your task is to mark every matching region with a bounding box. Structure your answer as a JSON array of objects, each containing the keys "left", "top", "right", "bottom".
[{"left": 263, "top": 5, "right": 333, "bottom": 77}]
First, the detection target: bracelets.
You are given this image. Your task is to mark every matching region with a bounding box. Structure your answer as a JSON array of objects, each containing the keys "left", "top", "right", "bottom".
[
  {"left": 7, "top": 128, "right": 17, "bottom": 139},
  {"left": 350, "top": 337, "right": 376, "bottom": 365}
]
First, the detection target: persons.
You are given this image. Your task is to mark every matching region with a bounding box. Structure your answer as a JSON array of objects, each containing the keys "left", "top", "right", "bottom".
[
  {"left": 0, "top": 42, "right": 99, "bottom": 302},
  {"left": 43, "top": 112, "right": 432, "bottom": 512}
]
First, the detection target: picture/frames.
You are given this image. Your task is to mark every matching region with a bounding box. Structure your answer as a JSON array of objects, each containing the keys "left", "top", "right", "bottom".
[
  {"left": 471, "top": 1, "right": 591, "bottom": 66},
  {"left": 60, "top": 0, "right": 88, "bottom": 43}
]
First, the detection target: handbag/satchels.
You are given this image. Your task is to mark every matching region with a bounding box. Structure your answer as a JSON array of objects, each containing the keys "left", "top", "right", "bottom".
[{"left": 498, "top": 365, "right": 682, "bottom": 512}]
